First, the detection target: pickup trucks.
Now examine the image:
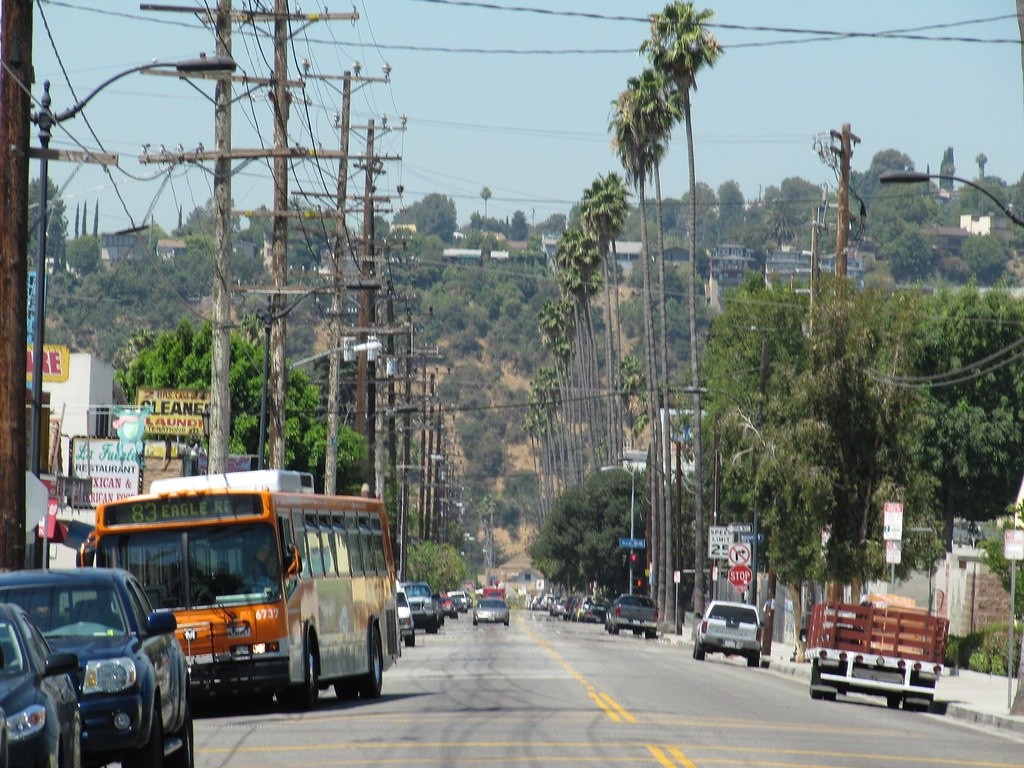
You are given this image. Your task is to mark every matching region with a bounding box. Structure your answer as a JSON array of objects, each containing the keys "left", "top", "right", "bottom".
[{"left": 692, "top": 598, "right": 764, "bottom": 668}]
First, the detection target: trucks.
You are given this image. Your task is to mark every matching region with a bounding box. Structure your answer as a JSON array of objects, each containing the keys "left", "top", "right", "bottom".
[{"left": 808, "top": 594, "right": 951, "bottom": 708}]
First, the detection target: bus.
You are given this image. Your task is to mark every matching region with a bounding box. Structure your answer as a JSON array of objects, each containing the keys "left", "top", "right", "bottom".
[{"left": 80, "top": 467, "right": 404, "bottom": 713}]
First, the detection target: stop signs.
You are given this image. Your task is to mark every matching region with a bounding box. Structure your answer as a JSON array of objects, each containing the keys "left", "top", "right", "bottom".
[{"left": 725, "top": 566, "right": 753, "bottom": 592}]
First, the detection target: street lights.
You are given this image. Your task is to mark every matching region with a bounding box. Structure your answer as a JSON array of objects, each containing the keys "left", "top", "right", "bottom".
[
  {"left": 368, "top": 404, "right": 418, "bottom": 501},
  {"left": 29, "top": 55, "right": 240, "bottom": 565},
  {"left": 685, "top": 384, "right": 758, "bottom": 601},
  {"left": 878, "top": 168, "right": 1023, "bottom": 231},
  {"left": 599, "top": 462, "right": 637, "bottom": 592},
  {"left": 254, "top": 278, "right": 381, "bottom": 474}
]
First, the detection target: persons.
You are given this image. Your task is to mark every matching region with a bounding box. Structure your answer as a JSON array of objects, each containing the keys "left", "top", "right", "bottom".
[
  {"left": 209, "top": 561, "right": 236, "bottom": 594},
  {"left": 240, "top": 560, "right": 275, "bottom": 593}
]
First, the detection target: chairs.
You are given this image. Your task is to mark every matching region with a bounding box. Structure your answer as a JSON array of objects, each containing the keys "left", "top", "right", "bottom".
[{"left": 72, "top": 600, "right": 112, "bottom": 624}]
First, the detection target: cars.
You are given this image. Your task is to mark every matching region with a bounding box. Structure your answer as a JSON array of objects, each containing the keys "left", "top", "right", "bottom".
[
  {"left": 528, "top": 591, "right": 605, "bottom": 624},
  {"left": 605, "top": 593, "right": 659, "bottom": 639},
  {"left": 0, "top": 568, "right": 195, "bottom": 768},
  {"left": 0, "top": 603, "right": 84, "bottom": 768},
  {"left": 395, "top": 578, "right": 415, "bottom": 647},
  {"left": 401, "top": 581, "right": 470, "bottom": 634},
  {"left": 473, "top": 599, "right": 512, "bottom": 628}
]
